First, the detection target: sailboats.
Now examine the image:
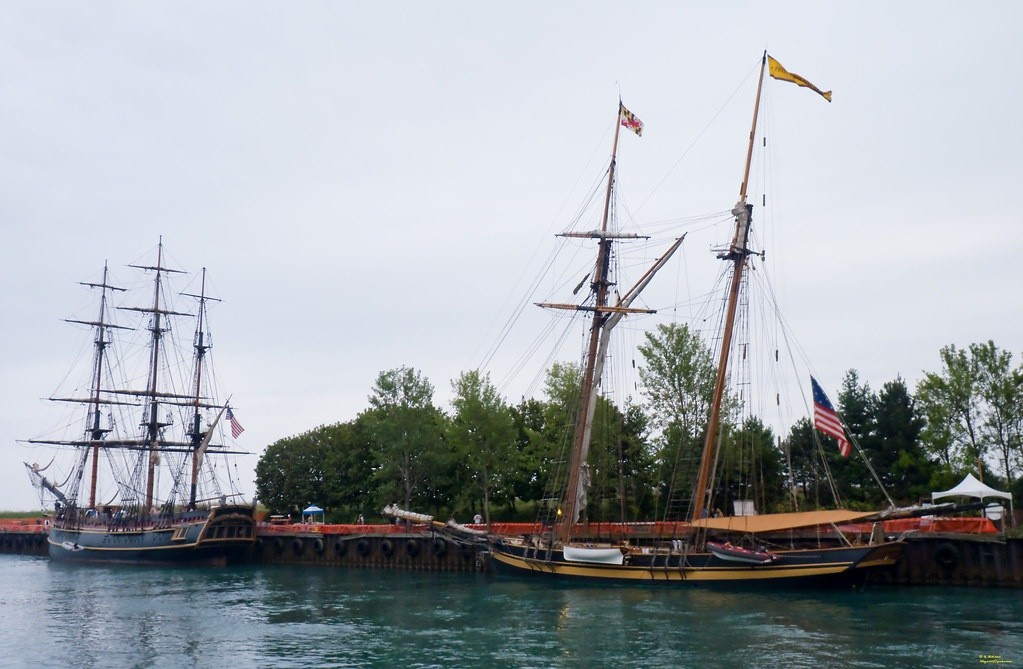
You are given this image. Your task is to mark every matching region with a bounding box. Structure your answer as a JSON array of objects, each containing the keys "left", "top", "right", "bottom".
[
  {"left": 14, "top": 232, "right": 260, "bottom": 568},
  {"left": 380, "top": 45, "right": 989, "bottom": 590}
]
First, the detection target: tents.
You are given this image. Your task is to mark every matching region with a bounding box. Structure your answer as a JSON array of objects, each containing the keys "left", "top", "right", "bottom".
[
  {"left": 931, "top": 474, "right": 1015, "bottom": 534},
  {"left": 302, "top": 505, "right": 324, "bottom": 524}
]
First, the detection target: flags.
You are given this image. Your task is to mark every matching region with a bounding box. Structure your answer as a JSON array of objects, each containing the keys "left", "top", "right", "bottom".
[
  {"left": 767, "top": 54, "right": 832, "bottom": 103},
  {"left": 621, "top": 104, "right": 645, "bottom": 137},
  {"left": 810, "top": 375, "right": 851, "bottom": 457},
  {"left": 226, "top": 406, "right": 244, "bottom": 439}
]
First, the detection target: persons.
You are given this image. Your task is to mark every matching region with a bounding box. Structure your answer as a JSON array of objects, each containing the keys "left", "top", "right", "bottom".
[
  {"left": 112, "top": 509, "right": 129, "bottom": 518},
  {"left": 358, "top": 514, "right": 364, "bottom": 525},
  {"left": 521, "top": 534, "right": 535, "bottom": 547},
  {"left": 474, "top": 513, "right": 482, "bottom": 524},
  {"left": 35, "top": 518, "right": 41, "bottom": 525},
  {"left": 186, "top": 507, "right": 197, "bottom": 512},
  {"left": 709, "top": 508, "right": 723, "bottom": 518},
  {"left": 308, "top": 515, "right": 311, "bottom": 523},
  {"left": 149, "top": 507, "right": 161, "bottom": 515},
  {"left": 44, "top": 517, "right": 50, "bottom": 525}
]
